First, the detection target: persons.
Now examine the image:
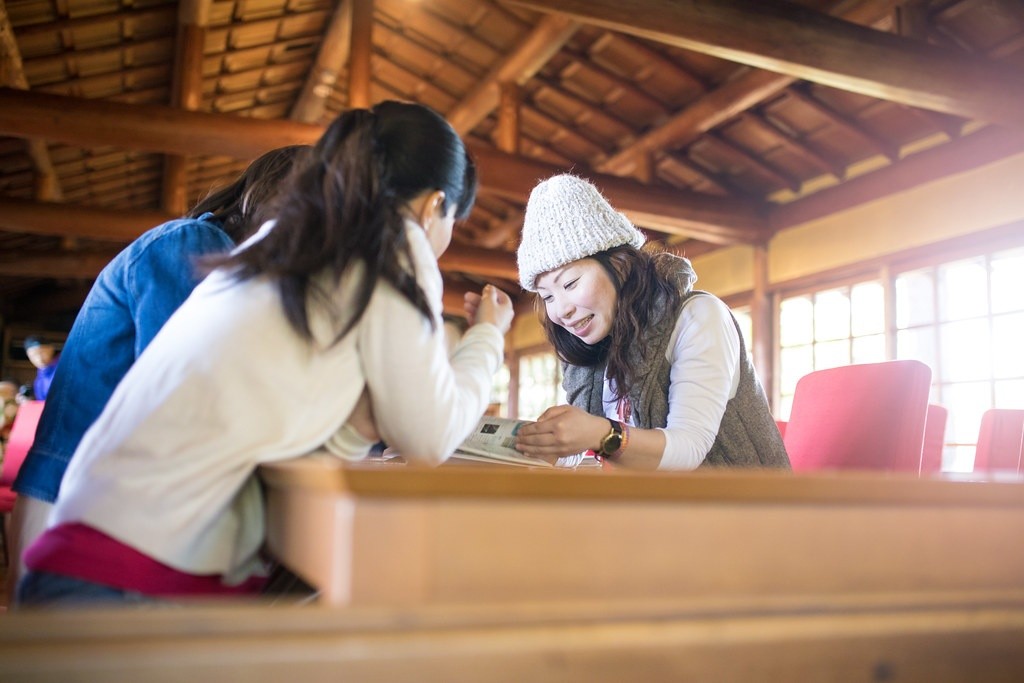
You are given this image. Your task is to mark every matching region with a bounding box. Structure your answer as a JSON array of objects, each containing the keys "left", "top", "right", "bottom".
[
  {"left": 510, "top": 171, "right": 793, "bottom": 472},
  {"left": 0, "top": 100, "right": 515, "bottom": 604}
]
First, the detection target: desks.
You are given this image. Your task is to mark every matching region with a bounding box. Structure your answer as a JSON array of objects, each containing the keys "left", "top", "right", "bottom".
[{"left": 265, "top": 455, "right": 1024, "bottom": 604}]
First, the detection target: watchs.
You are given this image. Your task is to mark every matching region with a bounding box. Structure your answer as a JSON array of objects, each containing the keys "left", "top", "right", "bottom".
[{"left": 596, "top": 417, "right": 625, "bottom": 459}]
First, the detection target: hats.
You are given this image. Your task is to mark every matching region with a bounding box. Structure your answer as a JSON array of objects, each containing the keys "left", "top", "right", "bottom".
[
  {"left": 516, "top": 173, "right": 647, "bottom": 293},
  {"left": 24, "top": 334, "right": 52, "bottom": 349}
]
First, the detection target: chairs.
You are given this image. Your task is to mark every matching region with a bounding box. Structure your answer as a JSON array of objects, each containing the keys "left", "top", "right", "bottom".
[{"left": 781, "top": 360, "right": 934, "bottom": 475}]
